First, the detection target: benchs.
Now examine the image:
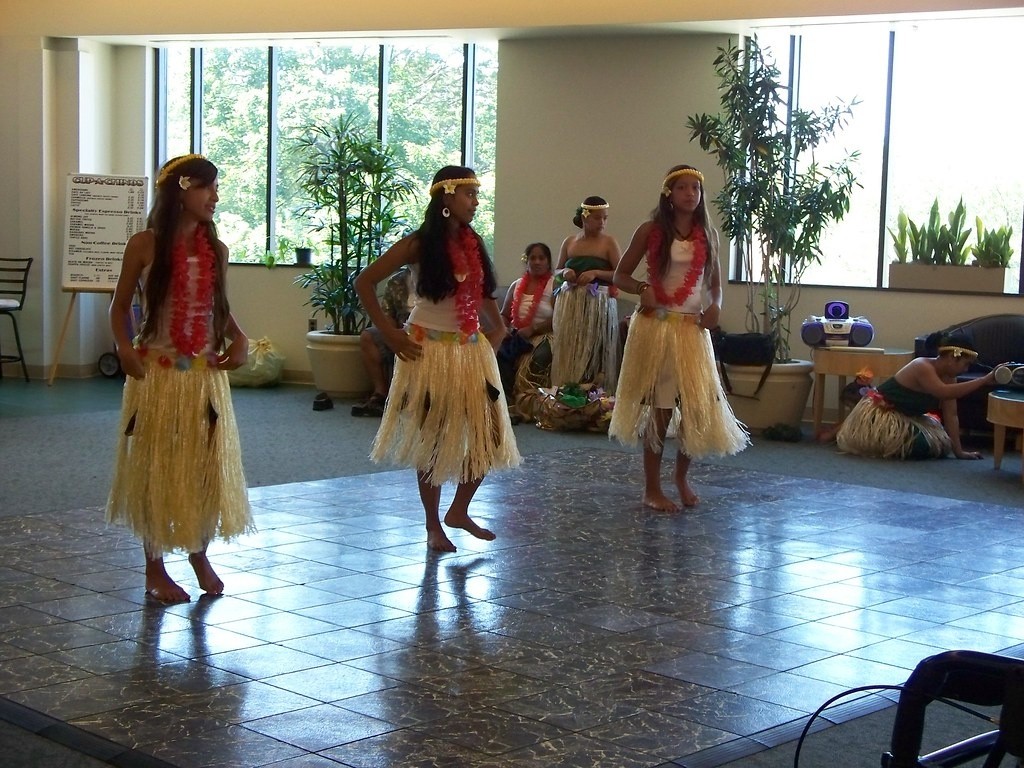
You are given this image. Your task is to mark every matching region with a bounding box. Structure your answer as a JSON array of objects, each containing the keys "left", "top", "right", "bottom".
[{"left": 912, "top": 312, "right": 1024, "bottom": 447}]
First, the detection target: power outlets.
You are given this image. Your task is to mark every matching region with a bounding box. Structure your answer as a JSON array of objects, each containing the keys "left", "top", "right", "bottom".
[{"left": 309, "top": 318, "right": 318, "bottom": 332}]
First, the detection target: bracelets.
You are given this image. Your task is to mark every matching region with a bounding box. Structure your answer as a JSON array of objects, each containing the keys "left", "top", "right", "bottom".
[{"left": 637, "top": 282, "right": 649, "bottom": 295}]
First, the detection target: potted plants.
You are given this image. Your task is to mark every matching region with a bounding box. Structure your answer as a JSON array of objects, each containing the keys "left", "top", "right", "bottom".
[
  {"left": 277, "top": 235, "right": 321, "bottom": 266},
  {"left": 288, "top": 110, "right": 421, "bottom": 399},
  {"left": 890, "top": 192, "right": 1014, "bottom": 293},
  {"left": 687, "top": 32, "right": 865, "bottom": 434}
]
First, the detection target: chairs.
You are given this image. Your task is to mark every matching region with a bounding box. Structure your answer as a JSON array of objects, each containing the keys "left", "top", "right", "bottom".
[{"left": 0, "top": 257, "right": 34, "bottom": 382}]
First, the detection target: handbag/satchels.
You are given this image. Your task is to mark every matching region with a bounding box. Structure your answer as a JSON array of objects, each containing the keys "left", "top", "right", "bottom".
[{"left": 716, "top": 333, "right": 775, "bottom": 366}]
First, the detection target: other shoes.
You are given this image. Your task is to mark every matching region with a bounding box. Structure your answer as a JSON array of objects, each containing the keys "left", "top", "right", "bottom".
[
  {"left": 313, "top": 392, "right": 331, "bottom": 411},
  {"left": 352, "top": 404, "right": 363, "bottom": 418},
  {"left": 363, "top": 393, "right": 385, "bottom": 415}
]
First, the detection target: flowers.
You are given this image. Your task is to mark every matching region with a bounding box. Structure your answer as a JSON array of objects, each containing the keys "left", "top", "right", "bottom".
[
  {"left": 582, "top": 209, "right": 591, "bottom": 217},
  {"left": 589, "top": 389, "right": 619, "bottom": 424},
  {"left": 522, "top": 253, "right": 528, "bottom": 262},
  {"left": 444, "top": 181, "right": 455, "bottom": 195}
]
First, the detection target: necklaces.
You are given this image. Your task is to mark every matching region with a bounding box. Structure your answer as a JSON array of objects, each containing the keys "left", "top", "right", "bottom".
[
  {"left": 448, "top": 226, "right": 485, "bottom": 337},
  {"left": 510, "top": 271, "right": 552, "bottom": 329},
  {"left": 170, "top": 225, "right": 216, "bottom": 356},
  {"left": 647, "top": 217, "right": 707, "bottom": 306},
  {"left": 673, "top": 224, "right": 693, "bottom": 243}
]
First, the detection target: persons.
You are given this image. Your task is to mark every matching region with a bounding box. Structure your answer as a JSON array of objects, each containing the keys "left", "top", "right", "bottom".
[
  {"left": 105, "top": 155, "right": 256, "bottom": 601},
  {"left": 496, "top": 243, "right": 554, "bottom": 424},
  {"left": 608, "top": 164, "right": 749, "bottom": 511},
  {"left": 358, "top": 233, "right": 416, "bottom": 414},
  {"left": 354, "top": 165, "right": 521, "bottom": 552},
  {"left": 821, "top": 335, "right": 1009, "bottom": 459},
  {"left": 552, "top": 197, "right": 622, "bottom": 395}
]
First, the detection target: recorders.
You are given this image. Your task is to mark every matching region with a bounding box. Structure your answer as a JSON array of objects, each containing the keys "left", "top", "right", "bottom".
[
  {"left": 994, "top": 361, "right": 1024, "bottom": 391},
  {"left": 801, "top": 302, "right": 874, "bottom": 347}
]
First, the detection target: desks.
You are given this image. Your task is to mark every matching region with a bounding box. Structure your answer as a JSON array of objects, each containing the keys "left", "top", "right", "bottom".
[
  {"left": 812, "top": 348, "right": 914, "bottom": 442},
  {"left": 986, "top": 390, "right": 1024, "bottom": 468}
]
